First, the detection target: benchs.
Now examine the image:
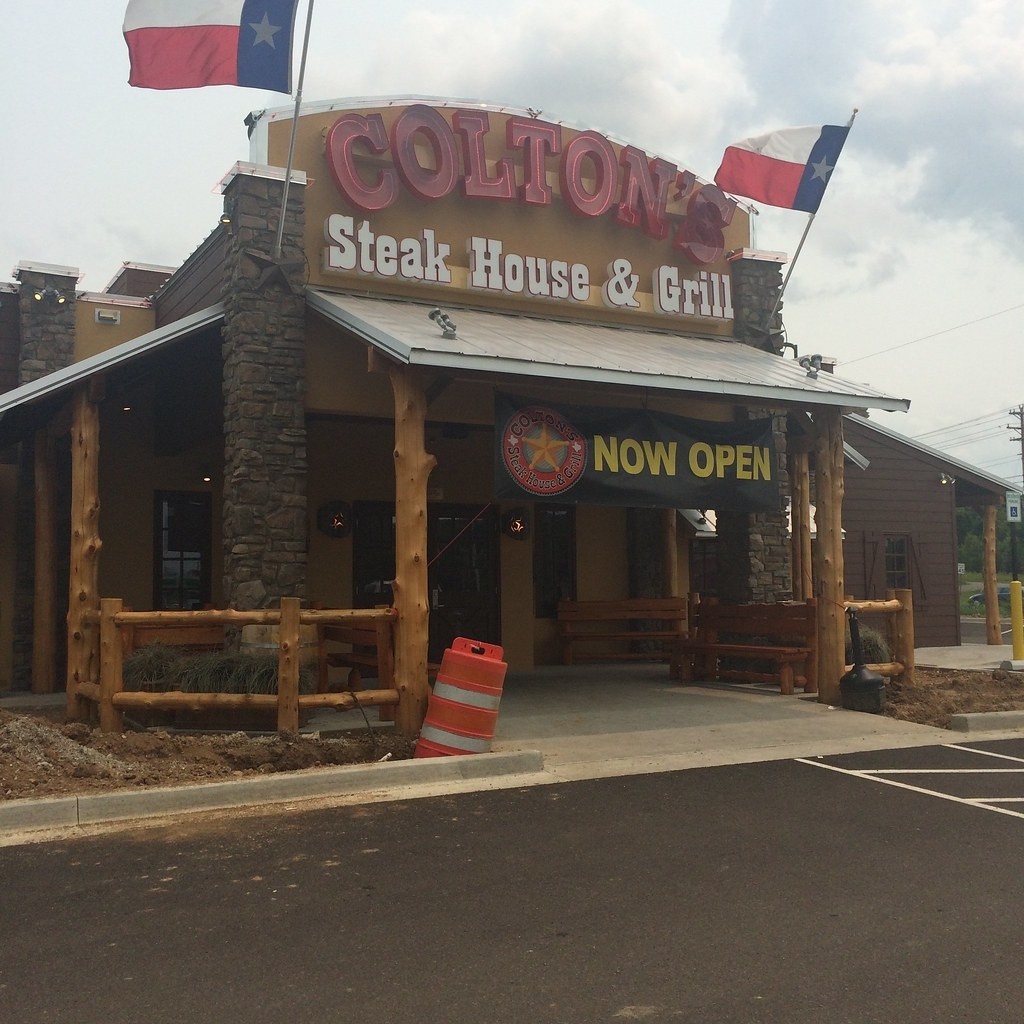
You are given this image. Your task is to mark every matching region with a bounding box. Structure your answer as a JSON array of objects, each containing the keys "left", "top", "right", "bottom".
[
  {"left": 325, "top": 620, "right": 442, "bottom": 689},
  {"left": 684, "top": 594, "right": 821, "bottom": 694},
  {"left": 555, "top": 595, "right": 687, "bottom": 666}
]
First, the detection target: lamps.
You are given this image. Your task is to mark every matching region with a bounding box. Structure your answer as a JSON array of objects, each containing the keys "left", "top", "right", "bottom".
[
  {"left": 427, "top": 307, "right": 458, "bottom": 339},
  {"left": 798, "top": 354, "right": 823, "bottom": 380},
  {"left": 50, "top": 289, "right": 66, "bottom": 304},
  {"left": 937, "top": 472, "right": 956, "bottom": 485},
  {"left": 94, "top": 308, "right": 121, "bottom": 325},
  {"left": 32, "top": 288, "right": 47, "bottom": 302}
]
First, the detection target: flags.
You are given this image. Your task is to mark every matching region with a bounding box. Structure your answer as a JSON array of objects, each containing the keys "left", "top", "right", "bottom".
[
  {"left": 713, "top": 126, "right": 848, "bottom": 214},
  {"left": 123, "top": 0, "right": 299, "bottom": 96}
]
